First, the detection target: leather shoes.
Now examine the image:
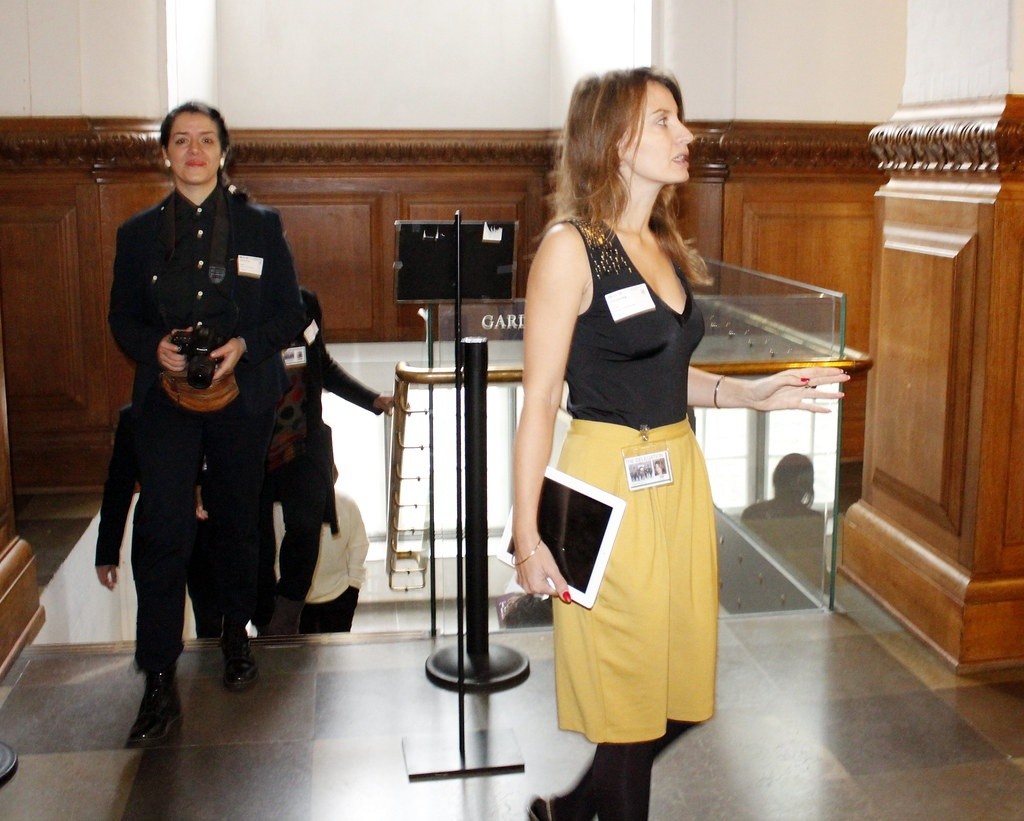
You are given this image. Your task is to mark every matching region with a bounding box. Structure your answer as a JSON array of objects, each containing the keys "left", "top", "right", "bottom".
[
  {"left": 126, "top": 673, "right": 182, "bottom": 747},
  {"left": 220, "top": 630, "right": 259, "bottom": 690}
]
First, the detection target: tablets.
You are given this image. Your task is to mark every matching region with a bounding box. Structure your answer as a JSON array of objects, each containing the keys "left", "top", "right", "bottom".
[{"left": 494, "top": 471, "right": 624, "bottom": 610}]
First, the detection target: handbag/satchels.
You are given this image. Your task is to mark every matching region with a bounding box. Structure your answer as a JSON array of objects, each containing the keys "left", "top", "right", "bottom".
[{"left": 161, "top": 366, "right": 240, "bottom": 413}]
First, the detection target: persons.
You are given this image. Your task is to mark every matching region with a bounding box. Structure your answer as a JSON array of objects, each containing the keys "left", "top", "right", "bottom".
[
  {"left": 736, "top": 453, "right": 831, "bottom": 593},
  {"left": 95, "top": 286, "right": 394, "bottom": 638},
  {"left": 654, "top": 459, "right": 665, "bottom": 475},
  {"left": 106, "top": 103, "right": 308, "bottom": 741},
  {"left": 630, "top": 463, "right": 653, "bottom": 481},
  {"left": 512, "top": 68, "right": 850, "bottom": 820}
]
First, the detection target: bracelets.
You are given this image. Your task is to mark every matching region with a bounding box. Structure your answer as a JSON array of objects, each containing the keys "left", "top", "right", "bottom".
[
  {"left": 236, "top": 336, "right": 249, "bottom": 353},
  {"left": 511, "top": 538, "right": 543, "bottom": 566},
  {"left": 713, "top": 375, "right": 726, "bottom": 409}
]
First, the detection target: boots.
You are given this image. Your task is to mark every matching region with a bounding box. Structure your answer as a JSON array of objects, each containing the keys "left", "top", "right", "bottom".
[{"left": 266, "top": 596, "right": 305, "bottom": 635}]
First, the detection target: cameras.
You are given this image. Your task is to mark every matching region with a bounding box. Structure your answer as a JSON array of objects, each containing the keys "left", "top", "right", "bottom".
[{"left": 172, "top": 322, "right": 229, "bottom": 388}]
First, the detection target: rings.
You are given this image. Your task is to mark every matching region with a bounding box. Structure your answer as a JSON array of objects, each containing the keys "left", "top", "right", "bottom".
[{"left": 804, "top": 384, "right": 818, "bottom": 389}]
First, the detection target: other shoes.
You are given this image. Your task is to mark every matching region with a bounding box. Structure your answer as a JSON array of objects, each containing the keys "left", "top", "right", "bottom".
[{"left": 527, "top": 794, "right": 562, "bottom": 821}]
